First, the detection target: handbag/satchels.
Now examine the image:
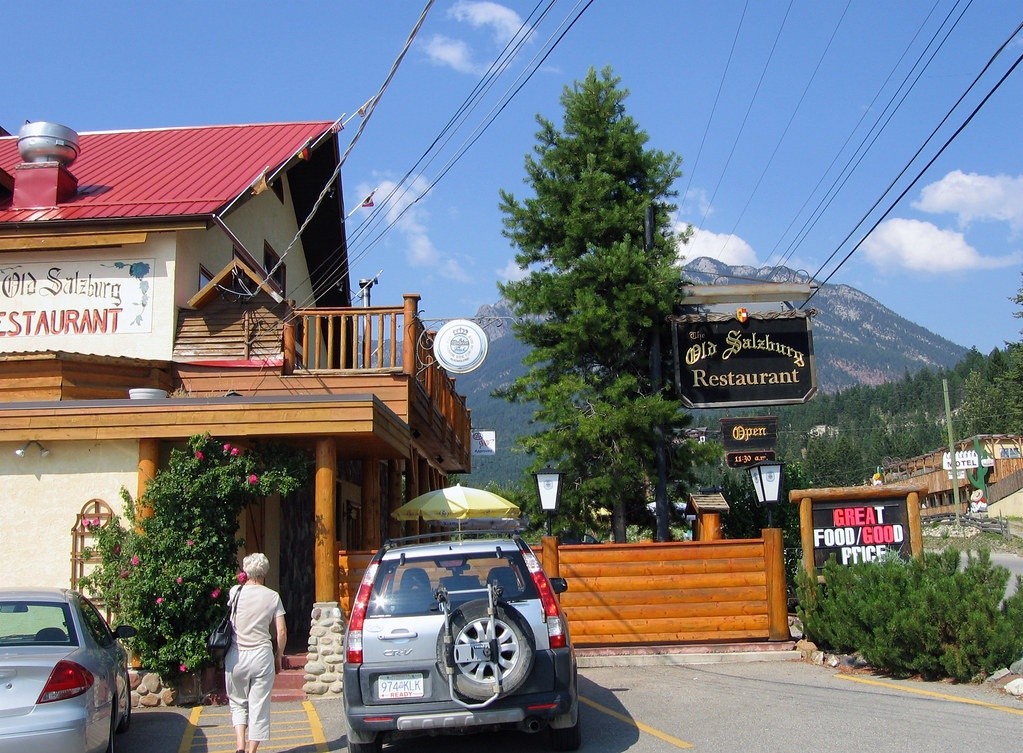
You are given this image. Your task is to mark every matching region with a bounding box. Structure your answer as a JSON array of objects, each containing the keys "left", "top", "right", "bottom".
[{"left": 206, "top": 583, "right": 245, "bottom": 659}]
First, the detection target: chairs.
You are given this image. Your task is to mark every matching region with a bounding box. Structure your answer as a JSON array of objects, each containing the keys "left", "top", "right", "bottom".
[
  {"left": 34, "top": 627, "right": 67, "bottom": 642},
  {"left": 485, "top": 566, "right": 524, "bottom": 598},
  {"left": 390, "top": 568, "right": 435, "bottom": 615}
]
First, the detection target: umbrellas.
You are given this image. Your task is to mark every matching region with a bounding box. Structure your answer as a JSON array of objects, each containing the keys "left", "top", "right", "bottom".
[{"left": 390, "top": 483, "right": 521, "bottom": 541}]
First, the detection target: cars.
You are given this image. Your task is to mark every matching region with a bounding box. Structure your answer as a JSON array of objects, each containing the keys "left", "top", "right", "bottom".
[{"left": 0, "top": 588, "right": 131, "bottom": 753}]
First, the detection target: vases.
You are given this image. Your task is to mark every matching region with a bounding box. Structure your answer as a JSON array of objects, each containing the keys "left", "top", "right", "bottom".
[{"left": 129, "top": 389, "right": 167, "bottom": 399}]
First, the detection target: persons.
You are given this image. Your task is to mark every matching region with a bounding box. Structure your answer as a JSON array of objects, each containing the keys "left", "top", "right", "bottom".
[
  {"left": 223, "top": 552, "right": 287, "bottom": 753},
  {"left": 969, "top": 490, "right": 988, "bottom": 516}
]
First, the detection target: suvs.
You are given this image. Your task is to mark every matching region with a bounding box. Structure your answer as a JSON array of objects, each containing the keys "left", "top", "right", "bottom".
[{"left": 343, "top": 526, "right": 580, "bottom": 753}]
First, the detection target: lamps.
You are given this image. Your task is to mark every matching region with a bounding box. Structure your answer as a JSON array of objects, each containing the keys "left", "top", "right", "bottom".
[
  {"left": 531, "top": 464, "right": 566, "bottom": 537},
  {"left": 16, "top": 440, "right": 32, "bottom": 458},
  {"left": 34, "top": 440, "right": 49, "bottom": 457},
  {"left": 744, "top": 455, "right": 786, "bottom": 528}
]
